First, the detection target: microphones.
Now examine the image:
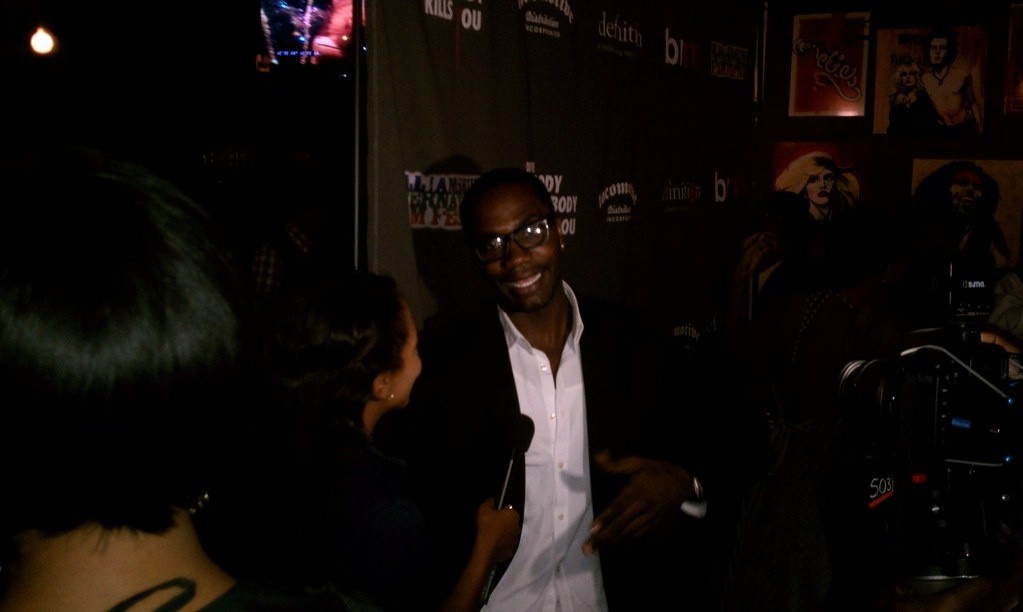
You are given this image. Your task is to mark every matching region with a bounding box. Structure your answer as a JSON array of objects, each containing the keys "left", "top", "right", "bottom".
[{"left": 495, "top": 415, "right": 535, "bottom": 508}]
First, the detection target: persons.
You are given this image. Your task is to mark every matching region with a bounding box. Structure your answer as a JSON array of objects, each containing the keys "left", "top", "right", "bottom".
[
  {"left": 414, "top": 166, "right": 717, "bottom": 612},
  {"left": 741, "top": 203, "right": 914, "bottom": 612},
  {"left": 0, "top": 147, "right": 375, "bottom": 612},
  {"left": 224, "top": 270, "right": 522, "bottom": 612}
]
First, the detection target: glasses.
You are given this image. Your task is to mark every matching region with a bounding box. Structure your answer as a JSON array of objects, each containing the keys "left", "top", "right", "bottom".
[{"left": 472, "top": 213, "right": 558, "bottom": 262}]
